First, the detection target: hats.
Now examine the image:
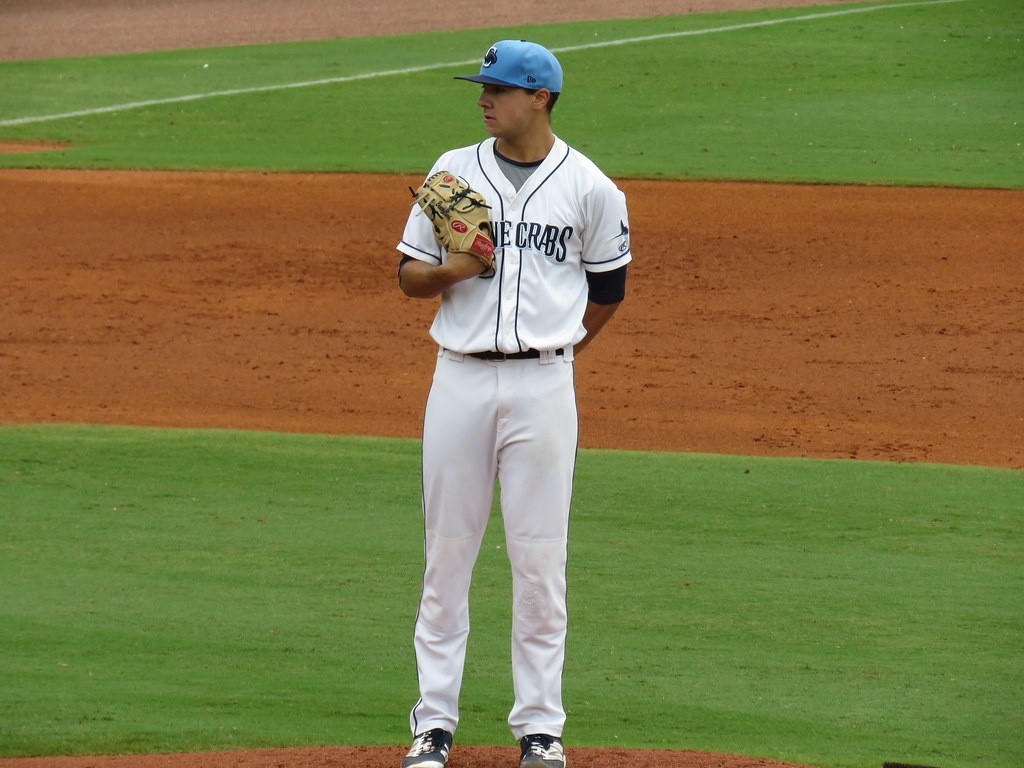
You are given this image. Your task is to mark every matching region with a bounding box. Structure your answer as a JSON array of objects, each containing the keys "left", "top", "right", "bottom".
[{"left": 453, "top": 38, "right": 563, "bottom": 92}]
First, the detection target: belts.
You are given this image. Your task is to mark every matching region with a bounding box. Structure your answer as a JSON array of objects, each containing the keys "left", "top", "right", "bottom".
[{"left": 443, "top": 348, "right": 563, "bottom": 360}]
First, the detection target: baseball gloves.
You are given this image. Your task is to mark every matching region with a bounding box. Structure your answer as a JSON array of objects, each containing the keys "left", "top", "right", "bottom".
[{"left": 408, "top": 170, "right": 504, "bottom": 283}]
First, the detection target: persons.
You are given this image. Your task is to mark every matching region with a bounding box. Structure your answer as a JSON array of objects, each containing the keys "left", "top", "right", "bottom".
[{"left": 395, "top": 39, "right": 633, "bottom": 768}]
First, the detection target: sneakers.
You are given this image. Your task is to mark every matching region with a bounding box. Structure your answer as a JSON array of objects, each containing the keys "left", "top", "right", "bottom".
[
  {"left": 520, "top": 736, "right": 567, "bottom": 768},
  {"left": 401, "top": 728, "right": 452, "bottom": 768}
]
